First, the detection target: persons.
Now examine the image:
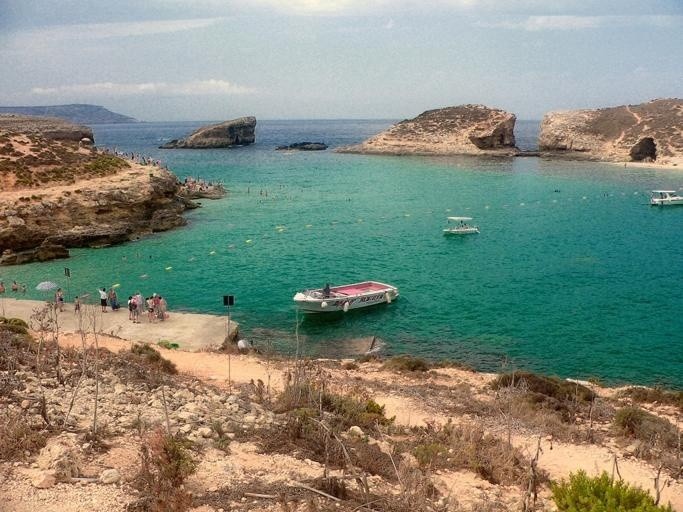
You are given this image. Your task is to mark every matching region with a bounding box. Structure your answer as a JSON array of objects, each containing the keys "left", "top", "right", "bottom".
[
  {"left": 54, "top": 288, "right": 64, "bottom": 312},
  {"left": 128, "top": 290, "right": 167, "bottom": 323},
  {"left": 115, "top": 151, "right": 160, "bottom": 166},
  {"left": 184, "top": 176, "right": 208, "bottom": 192},
  {"left": 96, "top": 287, "right": 118, "bottom": 313},
  {"left": 74, "top": 296, "right": 81, "bottom": 314},
  {"left": 0, "top": 280, "right": 26, "bottom": 293},
  {"left": 323, "top": 282, "right": 331, "bottom": 299}
]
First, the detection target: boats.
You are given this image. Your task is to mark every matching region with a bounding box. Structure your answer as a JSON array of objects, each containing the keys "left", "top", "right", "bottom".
[
  {"left": 442, "top": 217, "right": 480, "bottom": 234},
  {"left": 293, "top": 281, "right": 399, "bottom": 313},
  {"left": 650, "top": 190, "right": 683, "bottom": 205}
]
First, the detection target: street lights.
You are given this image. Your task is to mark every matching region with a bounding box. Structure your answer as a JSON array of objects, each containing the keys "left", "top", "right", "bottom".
[
  {"left": 65, "top": 267, "right": 71, "bottom": 302},
  {"left": 223, "top": 296, "right": 235, "bottom": 394}
]
